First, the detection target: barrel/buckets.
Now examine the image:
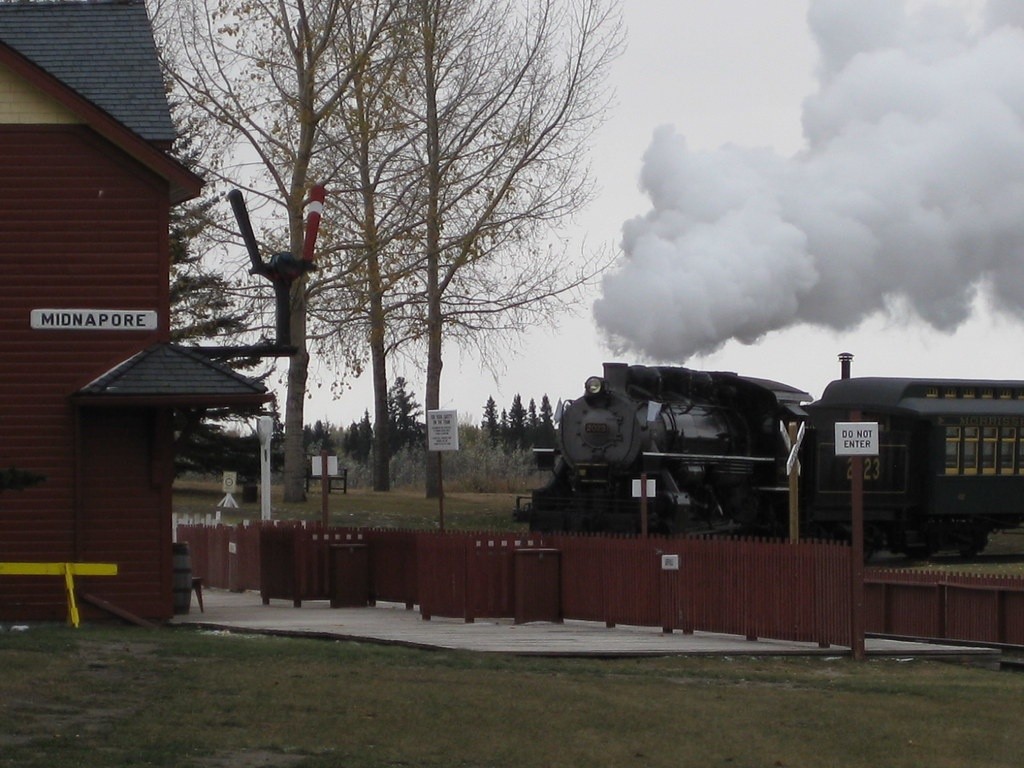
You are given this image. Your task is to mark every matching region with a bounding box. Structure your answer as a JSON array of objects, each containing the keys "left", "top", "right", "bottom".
[{"left": 173, "top": 541, "right": 193, "bottom": 615}]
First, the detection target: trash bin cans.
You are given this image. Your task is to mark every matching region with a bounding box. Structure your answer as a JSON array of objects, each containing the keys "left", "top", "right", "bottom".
[{"left": 174, "top": 541, "right": 192, "bottom": 615}]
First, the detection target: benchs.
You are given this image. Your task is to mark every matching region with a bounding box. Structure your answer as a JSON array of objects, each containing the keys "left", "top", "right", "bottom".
[{"left": 0, "top": 562, "right": 117, "bottom": 628}]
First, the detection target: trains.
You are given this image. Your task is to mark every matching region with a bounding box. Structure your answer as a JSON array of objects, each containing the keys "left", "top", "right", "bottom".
[{"left": 512, "top": 352, "right": 1024, "bottom": 563}]
